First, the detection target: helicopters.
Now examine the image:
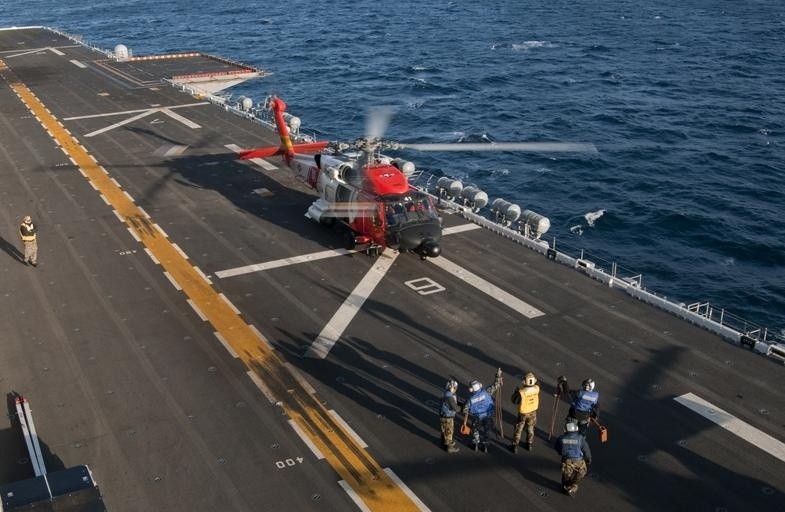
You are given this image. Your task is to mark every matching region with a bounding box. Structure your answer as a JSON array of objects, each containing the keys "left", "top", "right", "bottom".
[{"left": 241, "top": 97, "right": 597, "bottom": 259}]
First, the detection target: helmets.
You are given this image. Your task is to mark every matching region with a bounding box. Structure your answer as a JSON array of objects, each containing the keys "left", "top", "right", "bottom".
[
  {"left": 522, "top": 372, "right": 537, "bottom": 386},
  {"left": 582, "top": 378, "right": 595, "bottom": 391},
  {"left": 468, "top": 379, "right": 483, "bottom": 393},
  {"left": 445, "top": 380, "right": 458, "bottom": 391}
]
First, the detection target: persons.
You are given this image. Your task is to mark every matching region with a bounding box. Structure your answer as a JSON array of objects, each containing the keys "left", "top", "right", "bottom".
[
  {"left": 507, "top": 372, "right": 541, "bottom": 454},
  {"left": 438, "top": 379, "right": 462, "bottom": 454},
  {"left": 557, "top": 373, "right": 602, "bottom": 455},
  {"left": 555, "top": 421, "right": 594, "bottom": 500},
  {"left": 462, "top": 367, "right": 504, "bottom": 453},
  {"left": 409, "top": 199, "right": 427, "bottom": 212},
  {"left": 19, "top": 216, "right": 37, "bottom": 268}
]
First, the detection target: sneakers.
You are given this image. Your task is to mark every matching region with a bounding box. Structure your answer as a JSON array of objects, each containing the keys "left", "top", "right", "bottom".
[
  {"left": 445, "top": 438, "right": 533, "bottom": 454},
  {"left": 562, "top": 483, "right": 576, "bottom": 499}
]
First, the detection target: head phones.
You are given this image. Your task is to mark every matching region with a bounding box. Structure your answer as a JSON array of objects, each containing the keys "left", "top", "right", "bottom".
[
  {"left": 450, "top": 380, "right": 454, "bottom": 392},
  {"left": 467, "top": 381, "right": 483, "bottom": 393},
  {"left": 520, "top": 372, "right": 537, "bottom": 385}
]
